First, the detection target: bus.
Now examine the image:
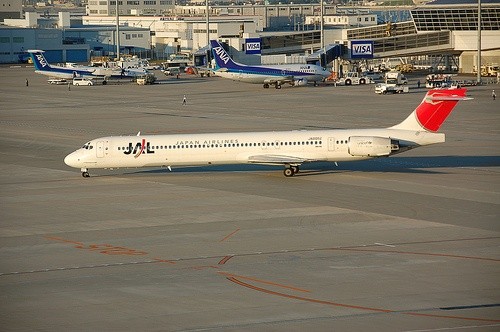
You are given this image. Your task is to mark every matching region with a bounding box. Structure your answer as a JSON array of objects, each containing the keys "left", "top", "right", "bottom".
[{"left": 160, "top": 62, "right": 181, "bottom": 71}]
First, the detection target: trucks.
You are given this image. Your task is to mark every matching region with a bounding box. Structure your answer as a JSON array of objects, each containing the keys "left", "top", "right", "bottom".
[{"left": 374, "top": 71, "right": 409, "bottom": 95}]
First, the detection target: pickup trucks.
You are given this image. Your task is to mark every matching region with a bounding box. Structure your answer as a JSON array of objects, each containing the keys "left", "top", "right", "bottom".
[{"left": 337, "top": 72, "right": 371, "bottom": 86}]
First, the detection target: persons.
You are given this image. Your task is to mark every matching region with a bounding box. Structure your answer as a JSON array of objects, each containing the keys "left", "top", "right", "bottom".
[
  {"left": 492, "top": 90, "right": 497, "bottom": 100},
  {"left": 182, "top": 95, "right": 188, "bottom": 106},
  {"left": 25, "top": 78, "right": 29, "bottom": 87},
  {"left": 334, "top": 78, "right": 337, "bottom": 88},
  {"left": 176, "top": 72, "right": 179, "bottom": 79},
  {"left": 68, "top": 81, "right": 71, "bottom": 91},
  {"left": 418, "top": 80, "right": 420, "bottom": 88}
]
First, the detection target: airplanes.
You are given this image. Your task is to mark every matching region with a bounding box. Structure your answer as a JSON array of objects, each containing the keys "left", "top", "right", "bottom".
[
  {"left": 27, "top": 49, "right": 157, "bottom": 86},
  {"left": 208, "top": 39, "right": 334, "bottom": 90},
  {"left": 65, "top": 85, "right": 473, "bottom": 177}
]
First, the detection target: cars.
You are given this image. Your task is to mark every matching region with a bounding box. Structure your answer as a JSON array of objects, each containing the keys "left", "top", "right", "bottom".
[{"left": 146, "top": 65, "right": 160, "bottom": 70}]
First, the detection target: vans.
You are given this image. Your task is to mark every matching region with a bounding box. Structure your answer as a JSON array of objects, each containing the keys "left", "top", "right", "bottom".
[{"left": 73, "top": 77, "right": 93, "bottom": 86}]
